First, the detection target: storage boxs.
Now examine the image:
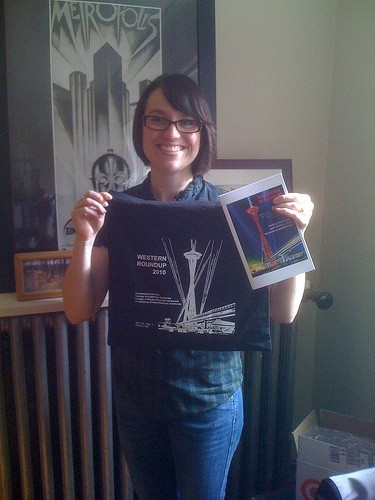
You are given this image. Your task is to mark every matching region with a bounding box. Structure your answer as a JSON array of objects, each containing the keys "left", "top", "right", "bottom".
[{"left": 291, "top": 408, "right": 375, "bottom": 500}]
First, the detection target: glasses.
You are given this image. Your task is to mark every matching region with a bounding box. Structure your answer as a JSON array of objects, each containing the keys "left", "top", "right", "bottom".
[{"left": 142, "top": 113, "right": 205, "bottom": 133}]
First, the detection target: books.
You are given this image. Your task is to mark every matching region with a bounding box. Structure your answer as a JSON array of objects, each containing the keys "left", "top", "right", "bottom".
[{"left": 218, "top": 173, "right": 316, "bottom": 292}]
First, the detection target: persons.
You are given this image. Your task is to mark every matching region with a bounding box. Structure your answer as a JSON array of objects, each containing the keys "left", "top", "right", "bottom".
[{"left": 61, "top": 73, "right": 315, "bottom": 500}]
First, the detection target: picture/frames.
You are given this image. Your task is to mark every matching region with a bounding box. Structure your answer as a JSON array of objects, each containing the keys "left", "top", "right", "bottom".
[
  {"left": 200, "top": 157, "right": 294, "bottom": 193},
  {"left": 14, "top": 249, "right": 74, "bottom": 302}
]
